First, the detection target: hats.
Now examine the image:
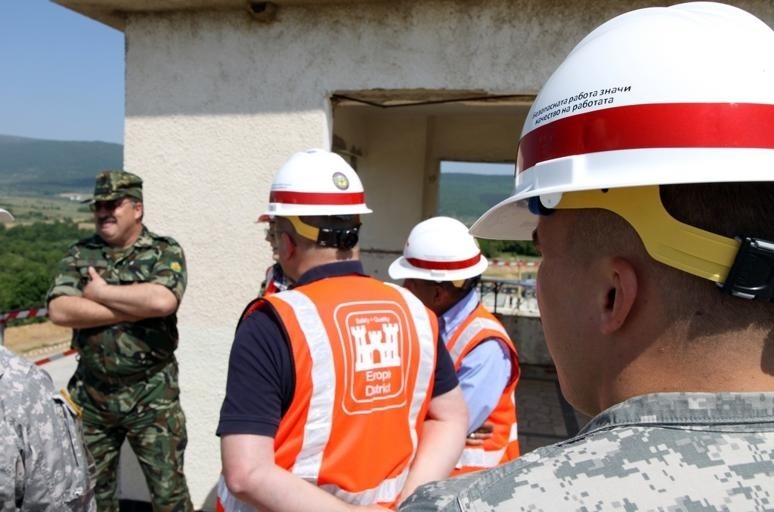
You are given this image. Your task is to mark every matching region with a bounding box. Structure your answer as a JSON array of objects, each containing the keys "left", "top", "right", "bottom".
[{"left": 94, "top": 172, "right": 142, "bottom": 201}]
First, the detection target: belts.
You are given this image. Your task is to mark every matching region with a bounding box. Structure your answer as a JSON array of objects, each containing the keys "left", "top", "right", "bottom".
[{"left": 80, "top": 356, "right": 173, "bottom": 386}]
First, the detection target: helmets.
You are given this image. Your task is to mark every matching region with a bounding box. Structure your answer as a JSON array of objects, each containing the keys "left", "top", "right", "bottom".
[
  {"left": 268, "top": 149, "right": 374, "bottom": 217},
  {"left": 467, "top": 2, "right": 774, "bottom": 241},
  {"left": 388, "top": 217, "right": 489, "bottom": 282}
]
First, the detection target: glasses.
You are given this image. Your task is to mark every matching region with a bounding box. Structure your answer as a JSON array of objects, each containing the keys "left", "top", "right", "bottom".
[{"left": 89, "top": 201, "right": 122, "bottom": 210}]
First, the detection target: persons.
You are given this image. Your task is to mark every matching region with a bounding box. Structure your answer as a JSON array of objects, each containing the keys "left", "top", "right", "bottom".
[
  {"left": 392, "top": 1, "right": 774, "bottom": 512},
  {"left": 257, "top": 217, "right": 297, "bottom": 298},
  {"left": 387, "top": 216, "right": 521, "bottom": 479},
  {"left": 215, "top": 148, "right": 468, "bottom": 512},
  {"left": 44, "top": 171, "right": 194, "bottom": 510},
  {"left": 0, "top": 208, "right": 99, "bottom": 511}
]
